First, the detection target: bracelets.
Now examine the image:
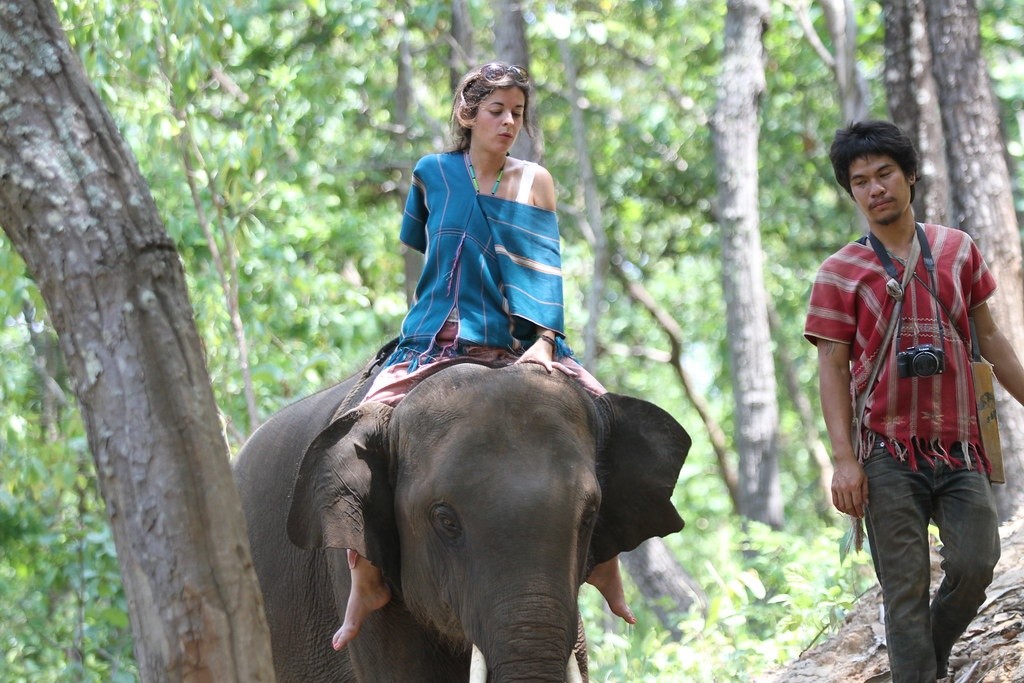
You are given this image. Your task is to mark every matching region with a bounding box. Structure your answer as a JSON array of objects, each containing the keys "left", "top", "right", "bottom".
[{"left": 537, "top": 333, "right": 557, "bottom": 348}]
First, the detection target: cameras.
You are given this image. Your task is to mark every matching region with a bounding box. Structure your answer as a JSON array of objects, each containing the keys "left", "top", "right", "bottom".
[{"left": 896, "top": 344, "right": 944, "bottom": 378}]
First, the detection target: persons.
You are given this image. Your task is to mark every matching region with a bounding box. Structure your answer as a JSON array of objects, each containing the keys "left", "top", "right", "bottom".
[
  {"left": 800, "top": 120, "right": 1024, "bottom": 683},
  {"left": 327, "top": 61, "right": 634, "bottom": 653}
]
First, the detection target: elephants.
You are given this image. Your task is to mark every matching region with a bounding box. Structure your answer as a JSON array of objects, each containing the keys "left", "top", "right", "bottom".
[{"left": 230, "top": 336, "right": 693, "bottom": 683}]
